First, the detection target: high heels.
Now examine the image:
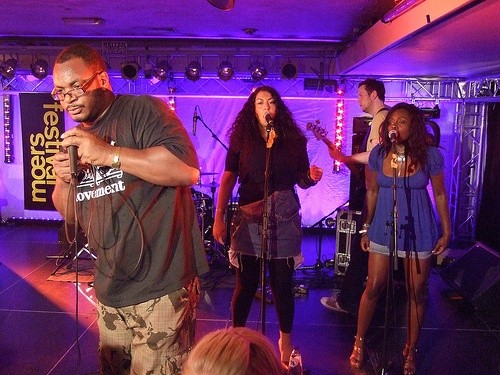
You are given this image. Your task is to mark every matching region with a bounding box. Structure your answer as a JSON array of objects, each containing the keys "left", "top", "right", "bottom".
[
  {"left": 279, "top": 340, "right": 290, "bottom": 369},
  {"left": 403, "top": 345, "right": 415, "bottom": 375},
  {"left": 350, "top": 338, "right": 366, "bottom": 367}
]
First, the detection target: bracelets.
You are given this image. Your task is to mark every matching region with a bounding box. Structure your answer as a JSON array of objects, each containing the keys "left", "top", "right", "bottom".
[
  {"left": 359, "top": 223, "right": 370, "bottom": 234},
  {"left": 112, "top": 146, "right": 121, "bottom": 170}
]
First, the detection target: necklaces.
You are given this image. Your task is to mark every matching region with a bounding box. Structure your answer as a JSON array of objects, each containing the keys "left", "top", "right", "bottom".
[{"left": 394, "top": 144, "right": 406, "bottom": 176}]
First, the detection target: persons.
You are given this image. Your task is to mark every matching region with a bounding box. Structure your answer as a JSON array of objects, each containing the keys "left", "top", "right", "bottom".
[
  {"left": 182, "top": 327, "right": 281, "bottom": 375},
  {"left": 51, "top": 45, "right": 209, "bottom": 375},
  {"left": 350, "top": 102, "right": 450, "bottom": 375},
  {"left": 212, "top": 86, "right": 323, "bottom": 368},
  {"left": 321, "top": 79, "right": 392, "bottom": 315}
]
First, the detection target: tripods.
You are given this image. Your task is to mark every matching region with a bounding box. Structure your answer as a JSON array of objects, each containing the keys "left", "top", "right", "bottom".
[{"left": 297, "top": 200, "right": 349, "bottom": 280}]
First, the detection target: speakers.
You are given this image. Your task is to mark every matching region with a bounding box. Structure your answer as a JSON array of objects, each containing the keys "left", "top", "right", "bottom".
[{"left": 456, "top": 241, "right": 500, "bottom": 316}]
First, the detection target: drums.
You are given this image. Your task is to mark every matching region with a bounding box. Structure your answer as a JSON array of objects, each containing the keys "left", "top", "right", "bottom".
[
  {"left": 228, "top": 197, "right": 240, "bottom": 222},
  {"left": 194, "top": 195, "right": 210, "bottom": 226}
]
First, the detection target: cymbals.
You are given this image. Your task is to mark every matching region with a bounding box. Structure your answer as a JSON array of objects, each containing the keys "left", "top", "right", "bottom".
[
  {"left": 202, "top": 182, "right": 220, "bottom": 187},
  {"left": 200, "top": 171, "right": 219, "bottom": 175}
]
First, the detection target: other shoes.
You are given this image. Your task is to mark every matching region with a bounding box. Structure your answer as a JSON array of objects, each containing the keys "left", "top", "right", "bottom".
[{"left": 320, "top": 296, "right": 346, "bottom": 311}]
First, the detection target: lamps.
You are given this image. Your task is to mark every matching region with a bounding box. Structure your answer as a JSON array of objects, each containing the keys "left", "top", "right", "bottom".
[
  {"left": 304, "top": 60, "right": 340, "bottom": 91},
  {"left": 0, "top": 58, "right": 17, "bottom": 78},
  {"left": 121, "top": 62, "right": 140, "bottom": 80},
  {"left": 279, "top": 56, "right": 300, "bottom": 80},
  {"left": 32, "top": 59, "right": 48, "bottom": 78},
  {"left": 187, "top": 60, "right": 203, "bottom": 80},
  {"left": 216, "top": 62, "right": 234, "bottom": 80},
  {"left": 156, "top": 60, "right": 170, "bottom": 79},
  {"left": 251, "top": 60, "right": 266, "bottom": 79}
]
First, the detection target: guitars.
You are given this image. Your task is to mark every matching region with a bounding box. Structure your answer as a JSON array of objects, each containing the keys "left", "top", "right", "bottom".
[{"left": 307, "top": 118, "right": 366, "bottom": 195}]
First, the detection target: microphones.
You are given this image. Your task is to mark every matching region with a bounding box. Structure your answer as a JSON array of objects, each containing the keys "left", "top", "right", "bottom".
[
  {"left": 193, "top": 106, "right": 197, "bottom": 132},
  {"left": 264, "top": 111, "right": 274, "bottom": 127},
  {"left": 67, "top": 145, "right": 78, "bottom": 180},
  {"left": 388, "top": 130, "right": 398, "bottom": 144}
]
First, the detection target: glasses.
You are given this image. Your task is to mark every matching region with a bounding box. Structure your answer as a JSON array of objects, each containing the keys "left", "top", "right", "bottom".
[{"left": 51, "top": 72, "right": 100, "bottom": 101}]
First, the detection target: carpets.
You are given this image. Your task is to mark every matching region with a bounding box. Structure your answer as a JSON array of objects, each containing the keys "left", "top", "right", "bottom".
[{"left": 46, "top": 256, "right": 236, "bottom": 288}]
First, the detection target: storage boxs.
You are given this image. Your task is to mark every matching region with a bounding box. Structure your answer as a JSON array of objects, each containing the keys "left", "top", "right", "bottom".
[{"left": 334, "top": 208, "right": 361, "bottom": 275}]
